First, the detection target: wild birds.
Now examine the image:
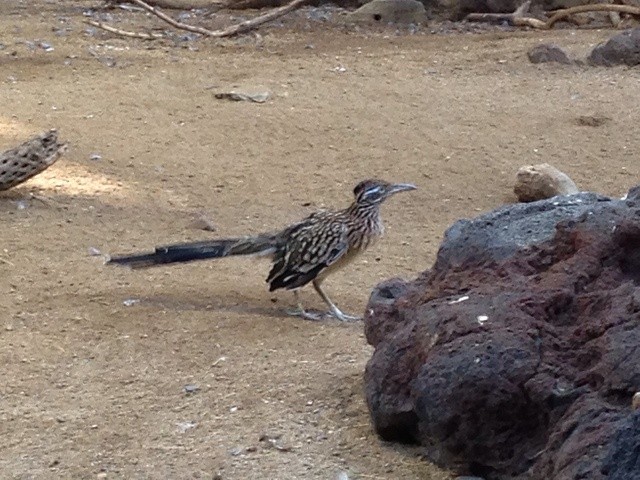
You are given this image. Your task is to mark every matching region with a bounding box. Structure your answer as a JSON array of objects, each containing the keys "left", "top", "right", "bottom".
[{"left": 102, "top": 178, "right": 420, "bottom": 322}]
